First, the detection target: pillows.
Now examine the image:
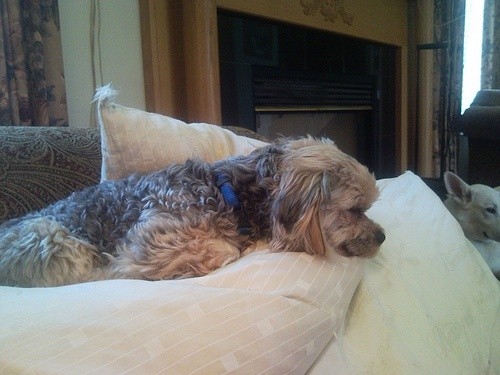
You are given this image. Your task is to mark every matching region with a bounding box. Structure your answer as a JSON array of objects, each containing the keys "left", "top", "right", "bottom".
[
  {"left": 0, "top": 125, "right": 103, "bottom": 221},
  {"left": 327, "top": 168, "right": 500, "bottom": 375},
  {"left": 94, "top": 86, "right": 276, "bottom": 186}
]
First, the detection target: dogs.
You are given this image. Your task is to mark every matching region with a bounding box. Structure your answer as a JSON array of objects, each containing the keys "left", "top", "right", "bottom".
[
  {"left": 1, "top": 134, "right": 388, "bottom": 290},
  {"left": 443, "top": 170, "right": 500, "bottom": 277}
]
